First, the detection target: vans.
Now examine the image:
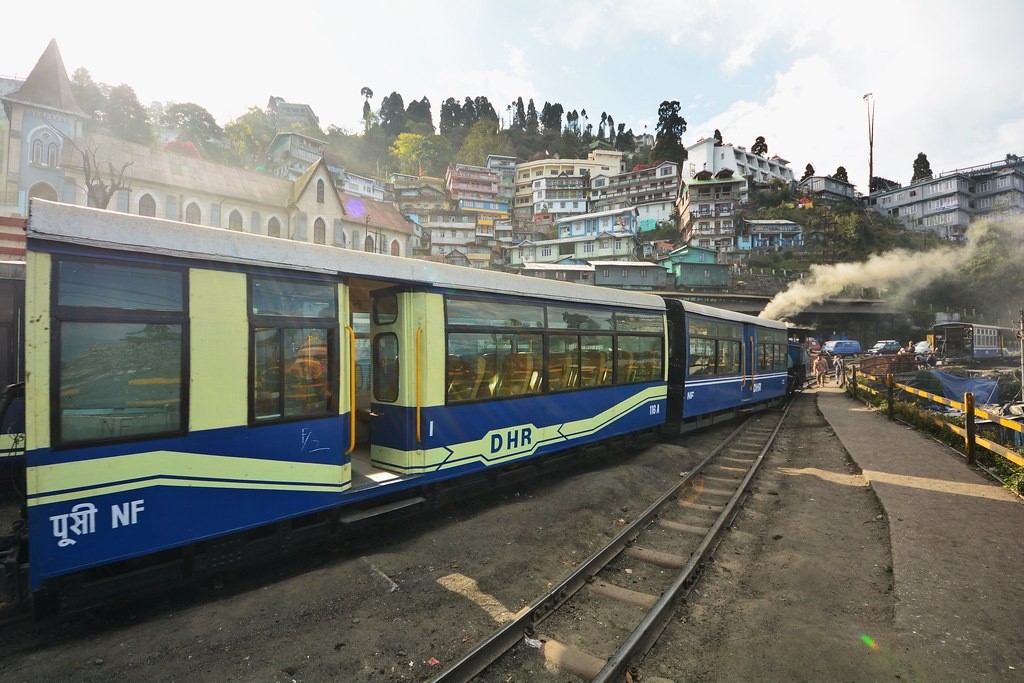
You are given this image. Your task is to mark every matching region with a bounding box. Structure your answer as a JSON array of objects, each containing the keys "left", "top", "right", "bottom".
[
  {"left": 806, "top": 337, "right": 821, "bottom": 352},
  {"left": 819, "top": 339, "right": 862, "bottom": 358}
]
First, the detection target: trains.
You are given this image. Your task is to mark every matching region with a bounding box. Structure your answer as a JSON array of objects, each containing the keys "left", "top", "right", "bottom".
[
  {"left": 926, "top": 321, "right": 1022, "bottom": 366},
  {"left": 0, "top": 194, "right": 818, "bottom": 611}
]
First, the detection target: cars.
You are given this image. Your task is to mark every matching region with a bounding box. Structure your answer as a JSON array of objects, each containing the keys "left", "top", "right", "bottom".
[
  {"left": 867, "top": 339, "right": 903, "bottom": 356},
  {"left": 912, "top": 340, "right": 931, "bottom": 357}
]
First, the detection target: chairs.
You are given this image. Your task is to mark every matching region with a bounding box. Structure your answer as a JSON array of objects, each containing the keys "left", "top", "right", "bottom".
[{"left": 444, "top": 353, "right": 738, "bottom": 404}]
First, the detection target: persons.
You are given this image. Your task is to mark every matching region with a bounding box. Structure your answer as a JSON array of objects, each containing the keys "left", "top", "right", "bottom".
[
  {"left": 897, "top": 341, "right": 940, "bottom": 370},
  {"left": 832, "top": 355, "right": 843, "bottom": 384},
  {"left": 813, "top": 354, "right": 828, "bottom": 388}
]
